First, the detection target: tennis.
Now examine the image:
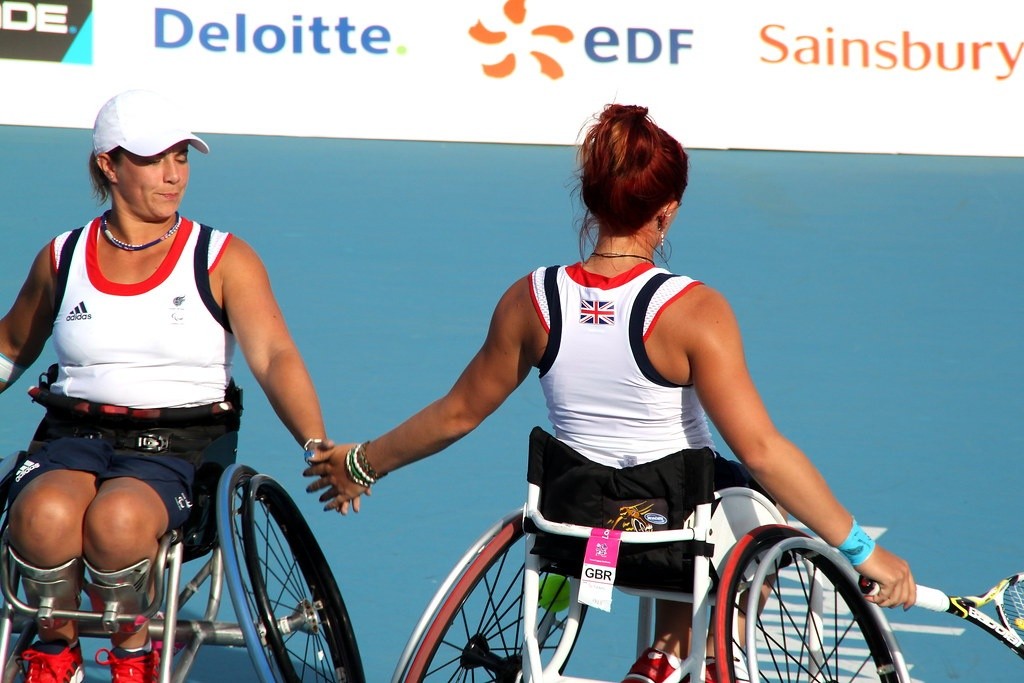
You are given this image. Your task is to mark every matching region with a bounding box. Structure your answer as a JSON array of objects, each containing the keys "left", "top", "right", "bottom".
[{"left": 539, "top": 575, "right": 568, "bottom": 609}]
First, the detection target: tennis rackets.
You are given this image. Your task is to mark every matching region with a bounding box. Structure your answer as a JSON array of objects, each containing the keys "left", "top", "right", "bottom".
[{"left": 858, "top": 569, "right": 1023, "bottom": 661}]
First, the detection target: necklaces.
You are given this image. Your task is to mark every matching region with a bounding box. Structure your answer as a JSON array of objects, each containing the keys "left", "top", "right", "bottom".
[
  {"left": 100, "top": 206, "right": 182, "bottom": 251},
  {"left": 589, "top": 249, "right": 657, "bottom": 268}
]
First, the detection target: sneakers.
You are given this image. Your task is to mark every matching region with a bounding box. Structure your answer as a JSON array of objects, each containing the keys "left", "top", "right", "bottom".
[
  {"left": 95, "top": 646, "right": 160, "bottom": 683},
  {"left": 22, "top": 638, "right": 85, "bottom": 683}
]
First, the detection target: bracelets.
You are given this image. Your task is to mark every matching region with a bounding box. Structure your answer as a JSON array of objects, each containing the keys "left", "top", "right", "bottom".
[
  {"left": 836, "top": 518, "right": 876, "bottom": 566},
  {"left": 345, "top": 439, "right": 388, "bottom": 488},
  {"left": 302, "top": 436, "right": 331, "bottom": 467}
]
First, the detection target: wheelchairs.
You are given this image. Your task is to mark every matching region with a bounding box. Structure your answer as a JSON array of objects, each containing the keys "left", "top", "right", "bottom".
[
  {"left": 389, "top": 426, "right": 915, "bottom": 683},
  {"left": 1, "top": 359, "right": 368, "bottom": 683}
]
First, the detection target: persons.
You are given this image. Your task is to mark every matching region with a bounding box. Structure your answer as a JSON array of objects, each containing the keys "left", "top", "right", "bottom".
[
  {"left": 0, "top": 87, "right": 371, "bottom": 683},
  {"left": 302, "top": 102, "right": 916, "bottom": 683}
]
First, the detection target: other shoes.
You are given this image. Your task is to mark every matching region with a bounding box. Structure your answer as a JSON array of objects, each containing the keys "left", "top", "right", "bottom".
[
  {"left": 705, "top": 657, "right": 750, "bottom": 683},
  {"left": 621, "top": 647, "right": 689, "bottom": 683}
]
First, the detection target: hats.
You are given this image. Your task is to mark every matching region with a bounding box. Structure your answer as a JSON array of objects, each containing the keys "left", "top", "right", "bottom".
[{"left": 92, "top": 91, "right": 210, "bottom": 157}]
